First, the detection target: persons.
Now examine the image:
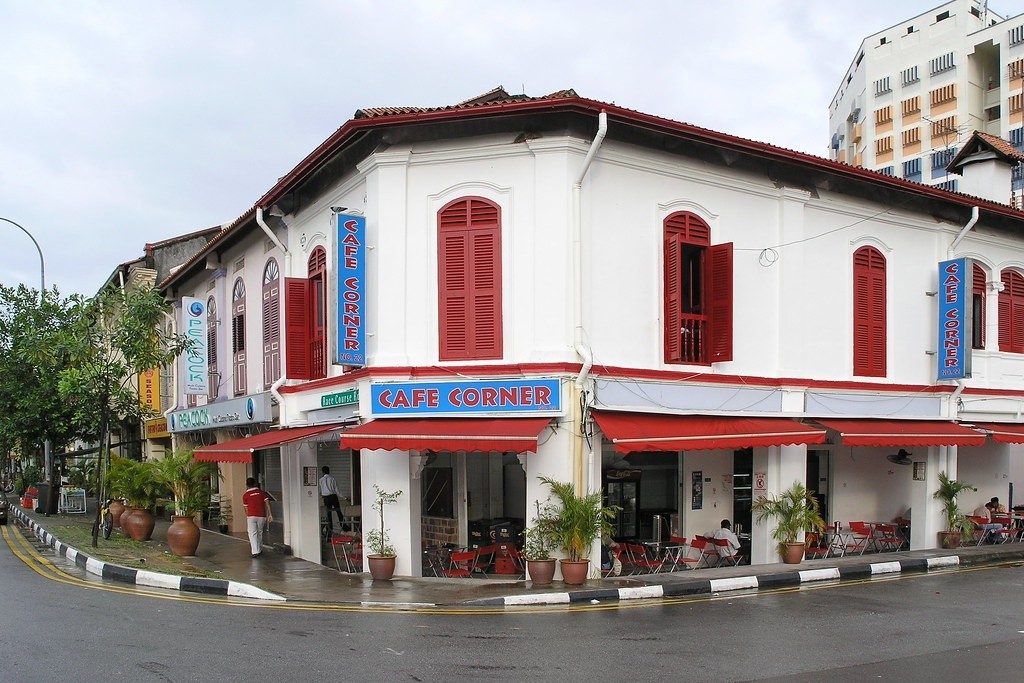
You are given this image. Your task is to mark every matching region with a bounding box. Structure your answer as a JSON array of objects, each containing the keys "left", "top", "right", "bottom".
[
  {"left": 714, "top": 519, "right": 751, "bottom": 564},
  {"left": 319, "top": 465, "right": 350, "bottom": 537},
  {"left": 974, "top": 497, "right": 1005, "bottom": 530},
  {"left": 601, "top": 529, "right": 616, "bottom": 570},
  {"left": 244, "top": 477, "right": 273, "bottom": 558}
]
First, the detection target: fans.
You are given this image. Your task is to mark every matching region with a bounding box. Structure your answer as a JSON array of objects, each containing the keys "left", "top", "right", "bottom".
[{"left": 887, "top": 449, "right": 912, "bottom": 465}]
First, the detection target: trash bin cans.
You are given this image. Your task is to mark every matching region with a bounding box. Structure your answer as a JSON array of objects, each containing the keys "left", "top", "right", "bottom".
[{"left": 35, "top": 480, "right": 63, "bottom": 515}]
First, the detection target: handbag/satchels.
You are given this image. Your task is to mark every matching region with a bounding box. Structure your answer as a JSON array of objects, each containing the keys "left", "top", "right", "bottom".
[{"left": 612, "top": 554, "right": 622, "bottom": 576}]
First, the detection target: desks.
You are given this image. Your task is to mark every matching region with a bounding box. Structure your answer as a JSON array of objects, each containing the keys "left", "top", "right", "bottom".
[
  {"left": 631, "top": 539, "right": 692, "bottom": 573},
  {"left": 820, "top": 530, "right": 859, "bottom": 556},
  {"left": 865, "top": 522, "right": 911, "bottom": 552},
  {"left": 991, "top": 515, "right": 1024, "bottom": 542}
]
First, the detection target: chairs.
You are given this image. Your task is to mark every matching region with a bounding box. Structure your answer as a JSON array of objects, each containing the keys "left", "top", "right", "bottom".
[{"left": 326, "top": 519, "right": 909, "bottom": 581}]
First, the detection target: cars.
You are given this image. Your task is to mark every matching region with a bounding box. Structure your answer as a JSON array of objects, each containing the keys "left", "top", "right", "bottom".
[{"left": 0, "top": 489, "right": 10, "bottom": 525}]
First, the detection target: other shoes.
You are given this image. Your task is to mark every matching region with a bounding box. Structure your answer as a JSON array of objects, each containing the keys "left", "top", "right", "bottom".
[{"left": 250, "top": 550, "right": 263, "bottom": 558}]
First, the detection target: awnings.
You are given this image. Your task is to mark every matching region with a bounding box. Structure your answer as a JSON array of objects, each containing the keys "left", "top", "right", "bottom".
[
  {"left": 193, "top": 423, "right": 343, "bottom": 465},
  {"left": 339, "top": 418, "right": 552, "bottom": 454},
  {"left": 814, "top": 418, "right": 987, "bottom": 448},
  {"left": 973, "top": 422, "right": 1024, "bottom": 444},
  {"left": 590, "top": 409, "right": 827, "bottom": 456}
]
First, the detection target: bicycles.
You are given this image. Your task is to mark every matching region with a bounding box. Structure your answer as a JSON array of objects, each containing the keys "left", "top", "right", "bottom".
[{"left": 91, "top": 499, "right": 113, "bottom": 540}]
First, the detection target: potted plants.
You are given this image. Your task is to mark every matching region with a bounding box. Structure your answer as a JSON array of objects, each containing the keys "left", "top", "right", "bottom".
[
  {"left": 367, "top": 484, "right": 403, "bottom": 578},
  {"left": 519, "top": 500, "right": 557, "bottom": 585},
  {"left": 536, "top": 475, "right": 622, "bottom": 585},
  {"left": 931, "top": 470, "right": 977, "bottom": 550},
  {"left": 753, "top": 482, "right": 824, "bottom": 563},
  {"left": 107, "top": 445, "right": 224, "bottom": 556}
]
